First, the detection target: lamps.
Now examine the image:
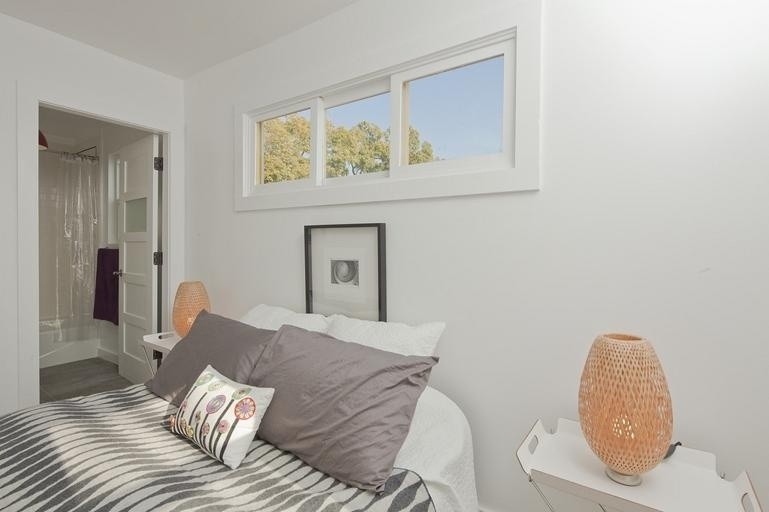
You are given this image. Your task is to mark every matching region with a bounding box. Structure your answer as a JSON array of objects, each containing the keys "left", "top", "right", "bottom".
[
  {"left": 173, "top": 281, "right": 212, "bottom": 341},
  {"left": 579, "top": 333, "right": 673, "bottom": 488},
  {"left": 39, "top": 128, "right": 48, "bottom": 151}
]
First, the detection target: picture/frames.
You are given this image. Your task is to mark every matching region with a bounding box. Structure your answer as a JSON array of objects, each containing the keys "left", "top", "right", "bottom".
[{"left": 304, "top": 223, "right": 388, "bottom": 321}]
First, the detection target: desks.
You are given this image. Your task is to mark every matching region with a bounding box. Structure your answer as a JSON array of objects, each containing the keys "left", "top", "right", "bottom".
[
  {"left": 515, "top": 416, "right": 763, "bottom": 512},
  {"left": 140, "top": 330, "right": 182, "bottom": 378}
]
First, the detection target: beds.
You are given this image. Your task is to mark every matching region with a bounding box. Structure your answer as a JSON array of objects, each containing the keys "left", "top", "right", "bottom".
[{"left": 1, "top": 304, "right": 471, "bottom": 511}]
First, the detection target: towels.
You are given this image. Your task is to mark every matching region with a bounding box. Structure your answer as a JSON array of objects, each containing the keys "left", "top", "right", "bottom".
[{"left": 93, "top": 248, "right": 119, "bottom": 326}]
